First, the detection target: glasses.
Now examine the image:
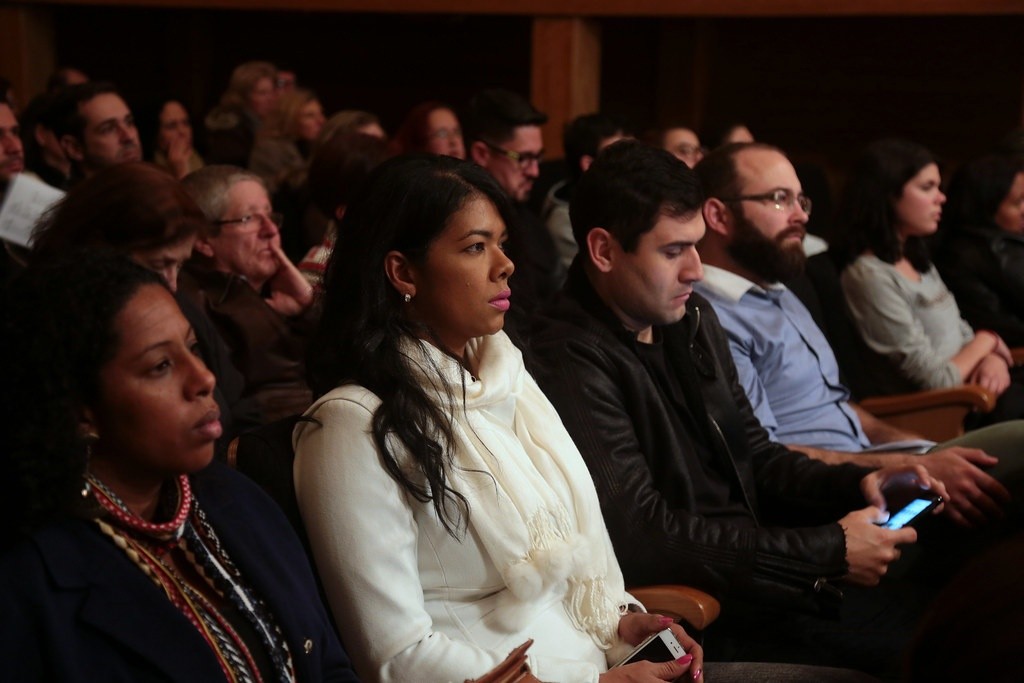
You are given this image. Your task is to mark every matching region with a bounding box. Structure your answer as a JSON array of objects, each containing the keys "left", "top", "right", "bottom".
[
  {"left": 420, "top": 129, "right": 462, "bottom": 141},
  {"left": 718, "top": 189, "right": 812, "bottom": 215},
  {"left": 210, "top": 212, "right": 283, "bottom": 231},
  {"left": 473, "top": 137, "right": 545, "bottom": 169}
]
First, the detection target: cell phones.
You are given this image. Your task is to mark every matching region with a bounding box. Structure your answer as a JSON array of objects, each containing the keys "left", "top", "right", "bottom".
[
  {"left": 880, "top": 491, "right": 944, "bottom": 530},
  {"left": 611, "top": 627, "right": 685, "bottom": 669}
]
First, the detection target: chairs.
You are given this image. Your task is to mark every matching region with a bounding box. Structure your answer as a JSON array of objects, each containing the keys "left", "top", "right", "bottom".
[
  {"left": 804, "top": 248, "right": 1024, "bottom": 444},
  {"left": 225, "top": 411, "right": 723, "bottom": 632}
]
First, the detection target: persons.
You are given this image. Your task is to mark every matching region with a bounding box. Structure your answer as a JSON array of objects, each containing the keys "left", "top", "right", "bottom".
[{"left": 0, "top": 62, "right": 1023, "bottom": 682}]
